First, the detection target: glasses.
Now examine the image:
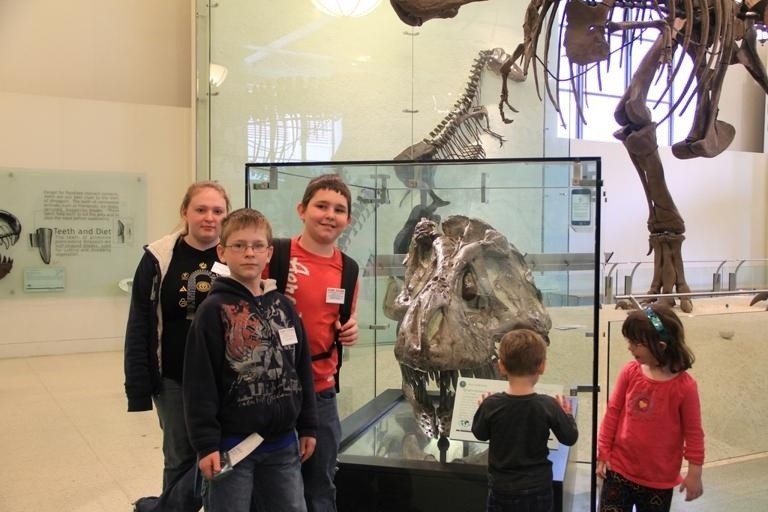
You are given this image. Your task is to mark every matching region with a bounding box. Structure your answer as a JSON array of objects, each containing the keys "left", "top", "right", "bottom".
[{"left": 223, "top": 240, "right": 271, "bottom": 254}]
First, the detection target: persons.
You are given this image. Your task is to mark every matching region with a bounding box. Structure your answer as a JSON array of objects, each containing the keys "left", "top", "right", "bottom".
[
  {"left": 122, "top": 179, "right": 231, "bottom": 512},
  {"left": 596, "top": 305, "right": 705, "bottom": 512},
  {"left": 471, "top": 327, "right": 578, "bottom": 512},
  {"left": 180, "top": 208, "right": 320, "bottom": 512},
  {"left": 259, "top": 174, "right": 361, "bottom": 512}
]
